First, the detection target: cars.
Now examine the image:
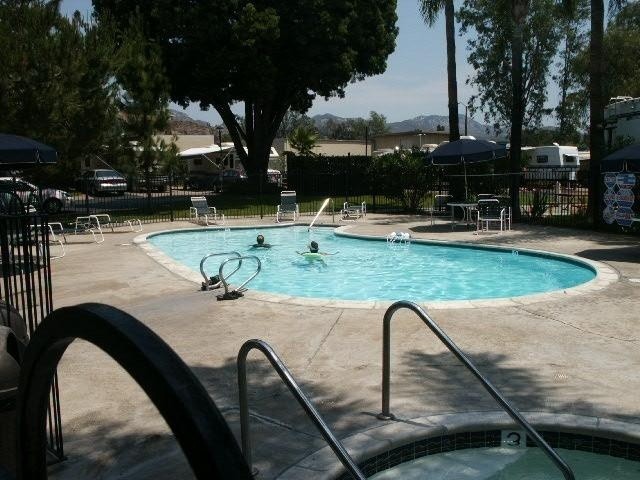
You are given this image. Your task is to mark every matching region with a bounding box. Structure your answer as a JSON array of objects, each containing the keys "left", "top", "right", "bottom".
[
  {"left": 0, "top": 176, "right": 74, "bottom": 214},
  {"left": 74, "top": 168, "right": 128, "bottom": 196}
]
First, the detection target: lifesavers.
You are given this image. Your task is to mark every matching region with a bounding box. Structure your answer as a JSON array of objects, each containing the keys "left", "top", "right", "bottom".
[
  {"left": 391, "top": 231, "right": 409, "bottom": 239},
  {"left": 43, "top": 197, "right": 62, "bottom": 215}
]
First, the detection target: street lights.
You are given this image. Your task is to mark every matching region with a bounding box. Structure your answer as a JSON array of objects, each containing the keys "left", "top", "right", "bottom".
[
  {"left": 458, "top": 100, "right": 468, "bottom": 136},
  {"left": 216, "top": 125, "right": 225, "bottom": 186}
]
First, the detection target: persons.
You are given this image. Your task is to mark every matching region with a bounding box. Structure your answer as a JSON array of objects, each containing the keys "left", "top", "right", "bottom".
[
  {"left": 294, "top": 240, "right": 340, "bottom": 271},
  {"left": 249, "top": 235, "right": 275, "bottom": 250}
]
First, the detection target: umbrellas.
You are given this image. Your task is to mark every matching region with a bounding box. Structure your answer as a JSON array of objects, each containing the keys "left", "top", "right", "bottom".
[
  {"left": 0, "top": 133, "right": 60, "bottom": 166},
  {"left": 422, "top": 138, "right": 508, "bottom": 200}
]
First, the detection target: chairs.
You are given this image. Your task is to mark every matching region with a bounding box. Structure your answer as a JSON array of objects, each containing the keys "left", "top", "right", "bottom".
[
  {"left": 276, "top": 191, "right": 300, "bottom": 223},
  {"left": 189, "top": 196, "right": 225, "bottom": 225},
  {"left": 8, "top": 214, "right": 143, "bottom": 260},
  {"left": 341, "top": 200, "right": 367, "bottom": 222},
  {"left": 431, "top": 193, "right": 513, "bottom": 231}
]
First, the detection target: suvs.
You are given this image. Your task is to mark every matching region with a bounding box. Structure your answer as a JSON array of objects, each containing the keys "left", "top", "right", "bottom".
[{"left": 133, "top": 156, "right": 169, "bottom": 193}]
